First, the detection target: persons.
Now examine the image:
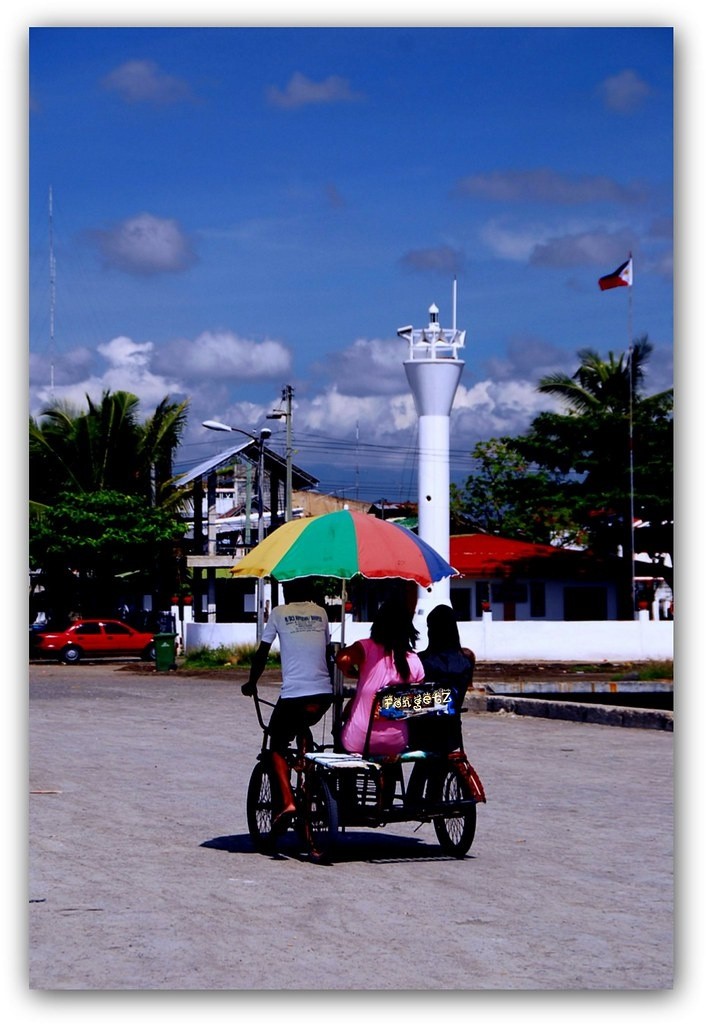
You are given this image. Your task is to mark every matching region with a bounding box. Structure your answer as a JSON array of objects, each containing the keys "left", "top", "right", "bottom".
[
  {"left": 405, "top": 605, "right": 476, "bottom": 812},
  {"left": 63, "top": 613, "right": 82, "bottom": 632},
  {"left": 336, "top": 602, "right": 426, "bottom": 813},
  {"left": 241, "top": 576, "right": 334, "bottom": 825}
]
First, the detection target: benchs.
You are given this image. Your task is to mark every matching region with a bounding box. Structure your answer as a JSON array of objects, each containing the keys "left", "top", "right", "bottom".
[{"left": 347, "top": 675, "right": 465, "bottom": 764}]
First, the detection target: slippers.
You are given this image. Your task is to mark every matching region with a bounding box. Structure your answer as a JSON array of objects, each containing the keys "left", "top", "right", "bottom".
[{"left": 272, "top": 809, "right": 294, "bottom": 825}]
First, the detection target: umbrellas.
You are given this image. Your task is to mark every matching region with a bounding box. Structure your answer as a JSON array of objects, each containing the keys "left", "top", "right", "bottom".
[{"left": 230, "top": 510, "right": 464, "bottom": 694}]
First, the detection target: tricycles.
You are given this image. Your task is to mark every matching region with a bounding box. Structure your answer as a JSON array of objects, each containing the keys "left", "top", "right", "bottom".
[{"left": 247, "top": 686, "right": 487, "bottom": 867}]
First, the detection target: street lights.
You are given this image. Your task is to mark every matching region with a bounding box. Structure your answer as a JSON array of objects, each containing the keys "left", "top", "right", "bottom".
[{"left": 200, "top": 420, "right": 272, "bottom": 646}]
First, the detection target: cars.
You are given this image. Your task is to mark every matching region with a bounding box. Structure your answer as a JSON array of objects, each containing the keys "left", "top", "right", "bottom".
[{"left": 34, "top": 619, "right": 158, "bottom": 665}]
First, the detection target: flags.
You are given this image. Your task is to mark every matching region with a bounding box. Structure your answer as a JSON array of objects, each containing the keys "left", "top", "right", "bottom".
[{"left": 598, "top": 260, "right": 632, "bottom": 291}]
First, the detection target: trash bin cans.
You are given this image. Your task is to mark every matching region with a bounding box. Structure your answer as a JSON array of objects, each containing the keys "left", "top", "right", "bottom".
[{"left": 153, "top": 632, "right": 179, "bottom": 673}]
